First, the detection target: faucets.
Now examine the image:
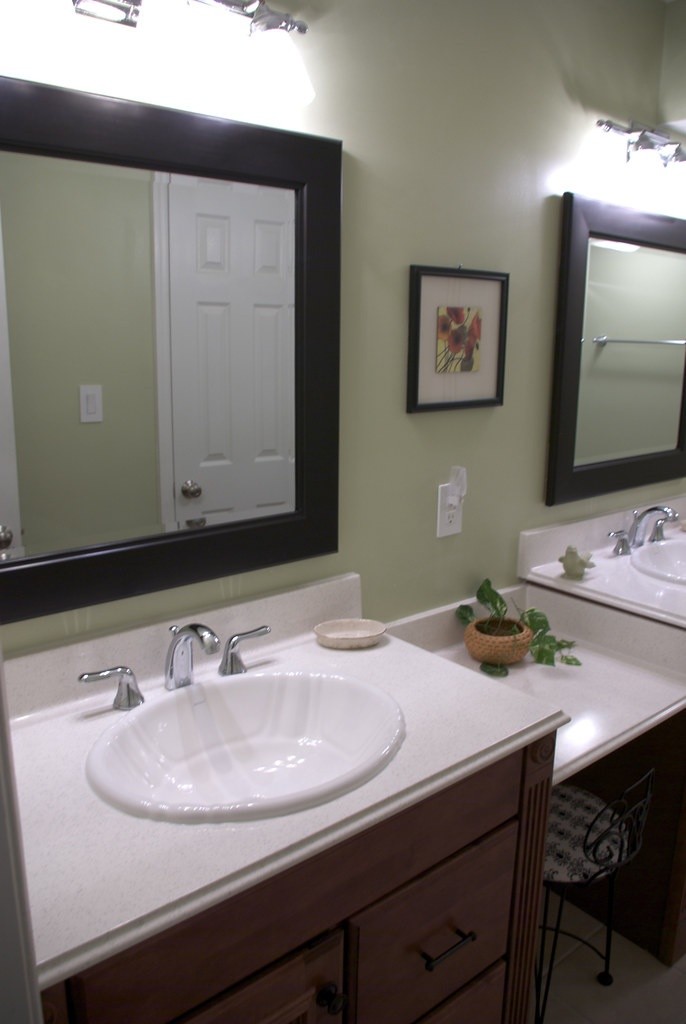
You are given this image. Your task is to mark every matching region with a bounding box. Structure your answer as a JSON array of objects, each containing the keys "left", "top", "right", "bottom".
[
  {"left": 624, "top": 505, "right": 680, "bottom": 548},
  {"left": 164, "top": 621, "right": 221, "bottom": 692}
]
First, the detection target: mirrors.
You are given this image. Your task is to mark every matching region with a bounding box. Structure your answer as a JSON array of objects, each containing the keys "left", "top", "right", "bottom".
[
  {"left": 0, "top": 73, "right": 342, "bottom": 626},
  {"left": 546, "top": 191, "right": 686, "bottom": 506}
]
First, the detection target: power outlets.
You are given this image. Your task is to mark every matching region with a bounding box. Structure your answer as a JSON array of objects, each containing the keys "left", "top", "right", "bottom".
[{"left": 437, "top": 483, "right": 462, "bottom": 539}]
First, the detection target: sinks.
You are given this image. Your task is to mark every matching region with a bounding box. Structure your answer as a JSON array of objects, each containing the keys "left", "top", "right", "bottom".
[
  {"left": 630, "top": 537, "right": 686, "bottom": 588},
  {"left": 84, "top": 671, "right": 408, "bottom": 824}
]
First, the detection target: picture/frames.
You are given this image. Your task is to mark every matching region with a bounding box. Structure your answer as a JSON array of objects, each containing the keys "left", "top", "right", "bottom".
[{"left": 406, "top": 264, "right": 510, "bottom": 414}]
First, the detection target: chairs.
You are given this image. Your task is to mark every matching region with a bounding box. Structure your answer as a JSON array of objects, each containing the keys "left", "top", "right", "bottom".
[{"left": 534, "top": 768, "right": 654, "bottom": 1024}]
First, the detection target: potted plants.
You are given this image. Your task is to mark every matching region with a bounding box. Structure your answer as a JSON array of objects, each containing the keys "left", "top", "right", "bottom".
[{"left": 455, "top": 578, "right": 582, "bottom": 677}]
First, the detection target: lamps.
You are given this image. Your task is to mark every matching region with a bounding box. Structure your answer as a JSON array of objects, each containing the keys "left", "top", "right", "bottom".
[
  {"left": 596, "top": 117, "right": 686, "bottom": 167},
  {"left": 70, "top": 0, "right": 307, "bottom": 36}
]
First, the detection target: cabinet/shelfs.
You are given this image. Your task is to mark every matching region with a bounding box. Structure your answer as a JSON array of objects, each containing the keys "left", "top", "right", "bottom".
[{"left": 40, "top": 722, "right": 556, "bottom": 1023}]
